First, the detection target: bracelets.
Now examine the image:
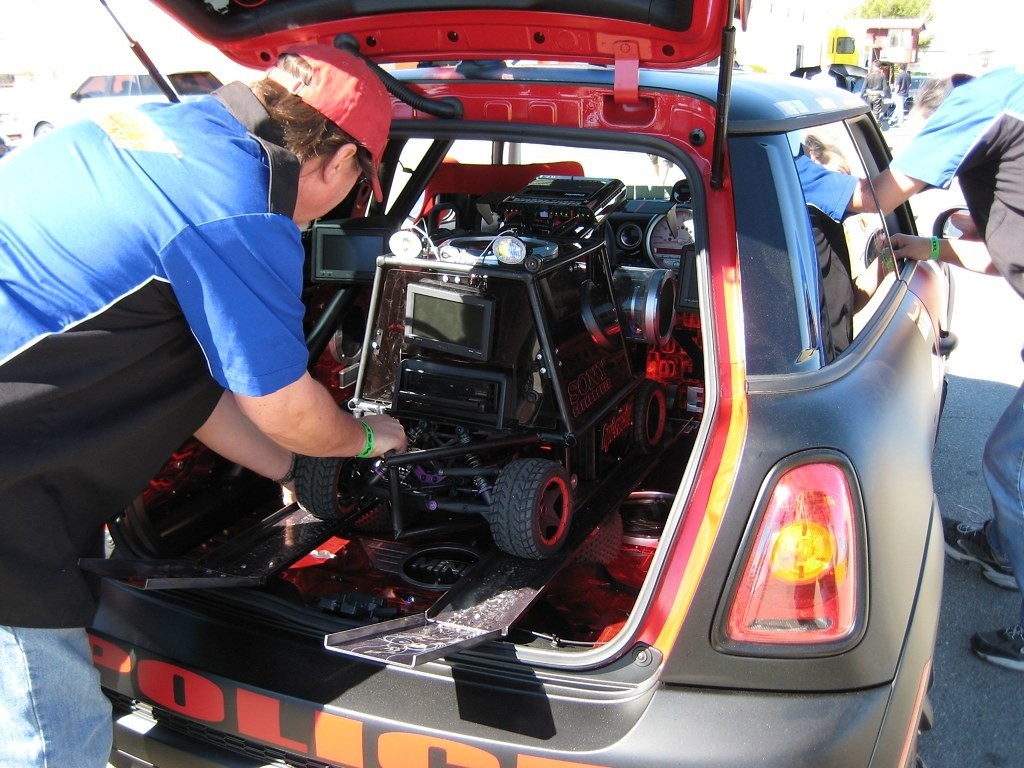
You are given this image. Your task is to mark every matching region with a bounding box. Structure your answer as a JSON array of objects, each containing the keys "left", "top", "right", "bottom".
[
  {"left": 353, "top": 420, "right": 374, "bottom": 458},
  {"left": 272, "top": 451, "right": 298, "bottom": 486},
  {"left": 930, "top": 236, "right": 939, "bottom": 260},
  {"left": 881, "top": 248, "right": 893, "bottom": 277}
]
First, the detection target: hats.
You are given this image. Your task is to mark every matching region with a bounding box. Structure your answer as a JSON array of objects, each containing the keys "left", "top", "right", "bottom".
[{"left": 268, "top": 45, "right": 391, "bottom": 204}]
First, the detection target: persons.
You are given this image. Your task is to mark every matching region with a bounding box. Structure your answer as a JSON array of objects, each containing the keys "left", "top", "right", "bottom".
[
  {"left": 793, "top": 134, "right": 879, "bottom": 359},
  {"left": 1, "top": 45, "right": 409, "bottom": 768},
  {"left": 860, "top": 60, "right": 887, "bottom": 124},
  {"left": 871, "top": 63, "right": 1024, "bottom": 674},
  {"left": 887, "top": 63, "right": 911, "bottom": 128}
]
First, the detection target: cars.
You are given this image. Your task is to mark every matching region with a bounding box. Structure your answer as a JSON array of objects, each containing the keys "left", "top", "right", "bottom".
[{"left": 64, "top": 0, "right": 969, "bottom": 768}]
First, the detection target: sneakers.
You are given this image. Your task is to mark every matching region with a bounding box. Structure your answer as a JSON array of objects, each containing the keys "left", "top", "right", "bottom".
[
  {"left": 940, "top": 517, "right": 1020, "bottom": 589},
  {"left": 971, "top": 624, "right": 1024, "bottom": 671}
]
